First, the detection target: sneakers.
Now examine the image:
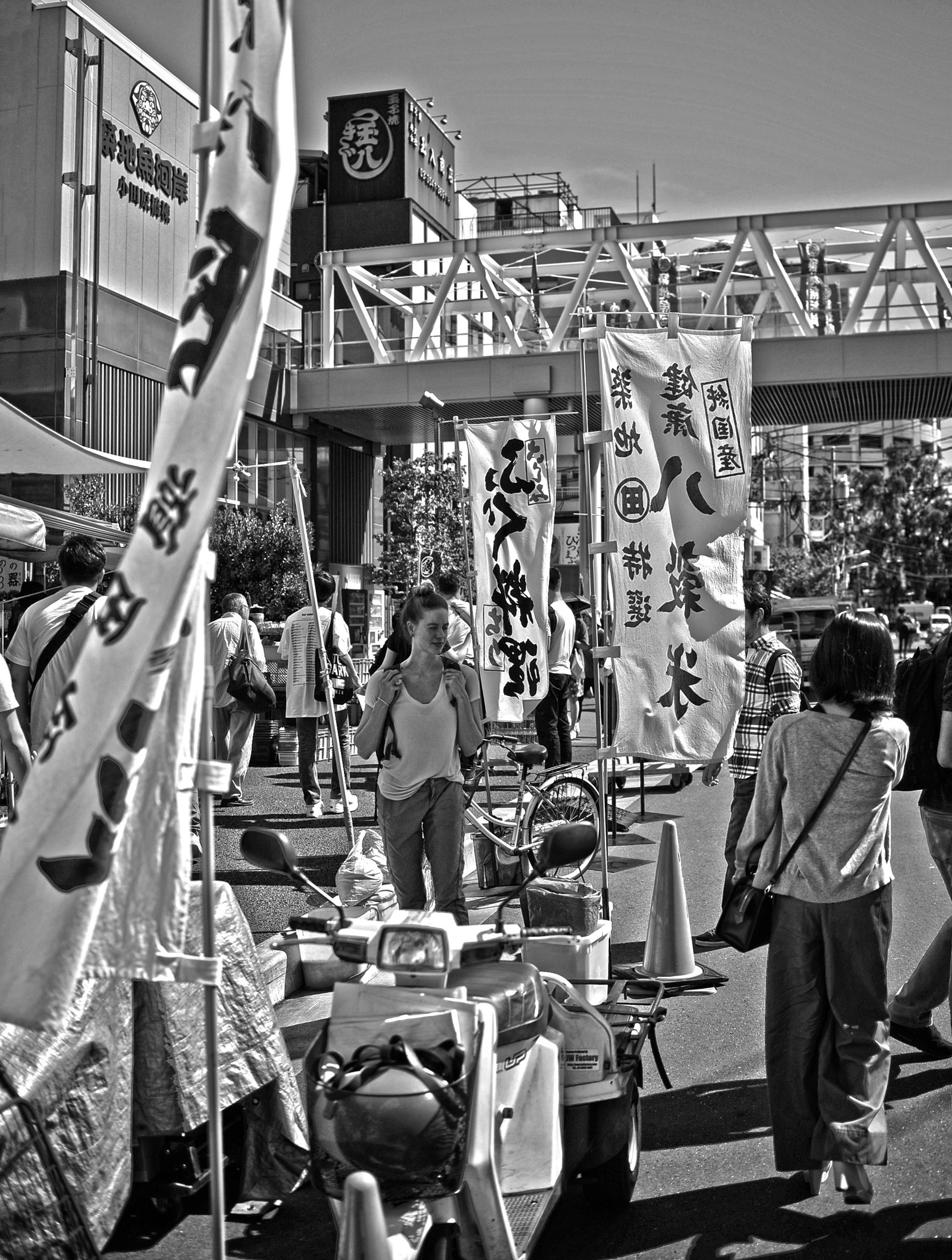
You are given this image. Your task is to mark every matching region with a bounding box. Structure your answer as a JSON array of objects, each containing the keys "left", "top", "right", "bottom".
[
  {"left": 305, "top": 800, "right": 325, "bottom": 819},
  {"left": 888, "top": 1019, "right": 952, "bottom": 1060},
  {"left": 696, "top": 926, "right": 730, "bottom": 947},
  {"left": 329, "top": 789, "right": 358, "bottom": 813}
]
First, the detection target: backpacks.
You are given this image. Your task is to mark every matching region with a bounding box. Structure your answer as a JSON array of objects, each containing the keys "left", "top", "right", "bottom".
[{"left": 887, "top": 644, "right": 940, "bottom": 793}]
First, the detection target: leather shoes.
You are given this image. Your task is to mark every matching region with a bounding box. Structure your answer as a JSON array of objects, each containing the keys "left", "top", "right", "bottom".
[{"left": 221, "top": 796, "right": 254, "bottom": 808}]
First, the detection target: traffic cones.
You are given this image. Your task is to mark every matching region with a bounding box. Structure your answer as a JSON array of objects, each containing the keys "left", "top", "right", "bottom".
[{"left": 612, "top": 821, "right": 732, "bottom": 995}]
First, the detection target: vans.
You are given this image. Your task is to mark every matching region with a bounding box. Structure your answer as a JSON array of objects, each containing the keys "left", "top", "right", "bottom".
[{"left": 928, "top": 613, "right": 952, "bottom": 635}]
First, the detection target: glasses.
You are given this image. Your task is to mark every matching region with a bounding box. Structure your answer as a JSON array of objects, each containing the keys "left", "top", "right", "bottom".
[{"left": 311, "top": 1041, "right": 392, "bottom": 1101}]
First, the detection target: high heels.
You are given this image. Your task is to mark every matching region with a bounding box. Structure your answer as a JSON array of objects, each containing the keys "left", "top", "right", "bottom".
[
  {"left": 832, "top": 1157, "right": 874, "bottom": 1206},
  {"left": 802, "top": 1158, "right": 833, "bottom": 1196}
]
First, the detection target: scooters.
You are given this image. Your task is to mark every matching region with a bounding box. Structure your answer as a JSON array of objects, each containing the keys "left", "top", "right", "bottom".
[{"left": 228, "top": 821, "right": 679, "bottom": 1260}]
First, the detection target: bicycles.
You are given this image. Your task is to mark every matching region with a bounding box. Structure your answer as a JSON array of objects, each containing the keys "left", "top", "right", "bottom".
[{"left": 455, "top": 732, "right": 604, "bottom": 885}]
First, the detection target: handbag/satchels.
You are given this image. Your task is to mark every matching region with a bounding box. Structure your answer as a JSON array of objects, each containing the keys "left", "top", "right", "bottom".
[
  {"left": 712, "top": 865, "right": 775, "bottom": 954},
  {"left": 226, "top": 649, "right": 277, "bottom": 715},
  {"left": 313, "top": 645, "right": 354, "bottom": 705}
]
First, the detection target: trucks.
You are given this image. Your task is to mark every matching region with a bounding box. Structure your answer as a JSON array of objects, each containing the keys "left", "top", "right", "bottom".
[
  {"left": 767, "top": 597, "right": 840, "bottom": 689},
  {"left": 892, "top": 600, "right": 952, "bottom": 630}
]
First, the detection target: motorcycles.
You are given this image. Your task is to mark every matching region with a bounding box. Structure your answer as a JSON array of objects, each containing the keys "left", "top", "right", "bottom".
[{"left": 928, "top": 632, "right": 943, "bottom": 648}]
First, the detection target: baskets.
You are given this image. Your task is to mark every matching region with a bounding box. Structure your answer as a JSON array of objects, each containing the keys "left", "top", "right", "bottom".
[
  {"left": 263, "top": 657, "right": 289, "bottom": 688},
  {"left": 301, "top": 1013, "right": 486, "bottom": 1202},
  {"left": 319, "top": 702, "right": 362, "bottom": 727},
  {"left": 248, "top": 720, "right": 299, "bottom": 767}
]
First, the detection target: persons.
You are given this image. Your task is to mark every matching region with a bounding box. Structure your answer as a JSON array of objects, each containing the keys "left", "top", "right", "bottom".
[
  {"left": 5, "top": 531, "right": 117, "bottom": 781},
  {"left": 731, "top": 609, "right": 916, "bottom": 1208},
  {"left": 275, "top": 570, "right": 365, "bottom": 820},
  {"left": 574, "top": 292, "right": 593, "bottom": 351},
  {"left": 197, "top": 529, "right": 952, "bottom": 703},
  {"left": 693, "top": 578, "right": 801, "bottom": 953},
  {"left": 351, "top": 587, "right": 489, "bottom": 930},
  {"left": 203, "top": 589, "right": 270, "bottom": 806},
  {"left": 523, "top": 564, "right": 583, "bottom": 794},
  {"left": 189, "top": 776, "right": 206, "bottom": 859},
  {"left": 619, "top": 298, "right": 634, "bottom": 329},
  {"left": 887, "top": 618, "right": 952, "bottom": 1067},
  {"left": 0, "top": 648, "right": 32, "bottom": 795}
]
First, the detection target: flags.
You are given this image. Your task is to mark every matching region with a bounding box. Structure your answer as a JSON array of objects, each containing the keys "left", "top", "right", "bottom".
[
  {"left": 650, "top": 254, "right": 680, "bottom": 330},
  {"left": 0, "top": 1, "right": 311, "bottom": 1039},
  {"left": 797, "top": 239, "right": 828, "bottom": 332},
  {"left": 462, "top": 417, "right": 567, "bottom": 724},
  {"left": 595, "top": 310, "right": 758, "bottom": 772}
]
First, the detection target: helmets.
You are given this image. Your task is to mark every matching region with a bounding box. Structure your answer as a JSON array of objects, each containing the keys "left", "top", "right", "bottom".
[{"left": 313, "top": 1060, "right": 459, "bottom": 1188}]
